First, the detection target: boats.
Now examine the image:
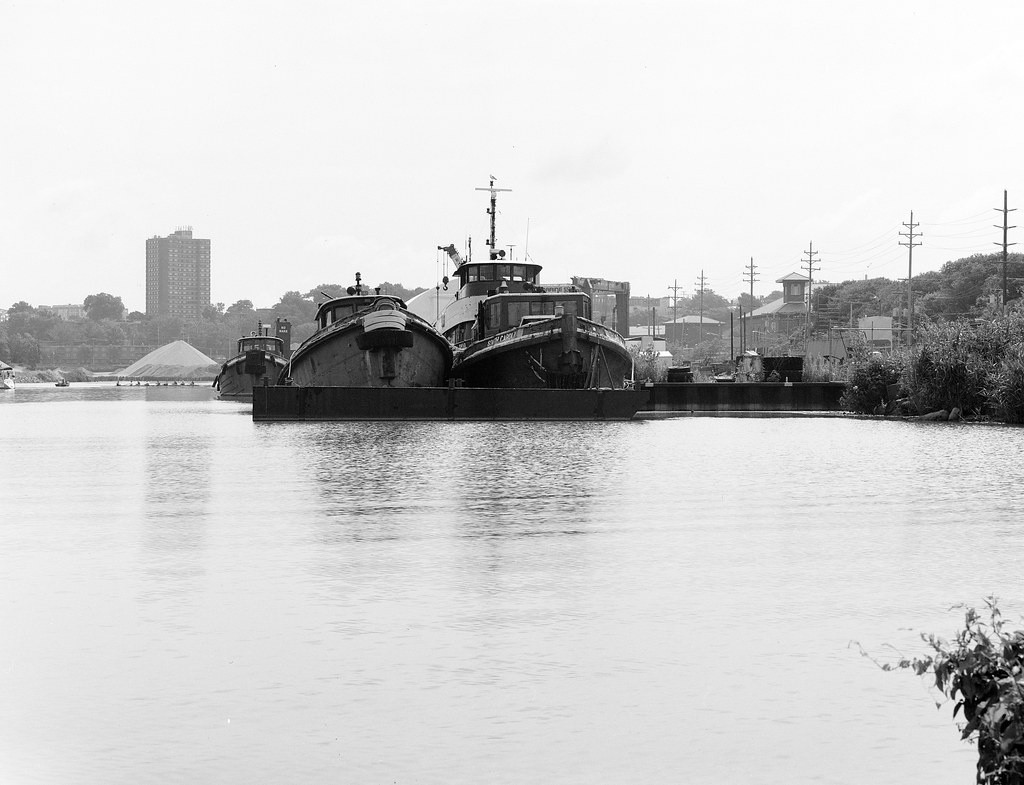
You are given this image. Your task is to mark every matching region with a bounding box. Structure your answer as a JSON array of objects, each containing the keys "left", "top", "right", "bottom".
[
  {"left": 714, "top": 374, "right": 737, "bottom": 382},
  {"left": 271, "top": 270, "right": 455, "bottom": 388},
  {"left": 55, "top": 381, "right": 70, "bottom": 387},
  {"left": 436, "top": 171, "right": 635, "bottom": 389},
  {"left": 213, "top": 318, "right": 291, "bottom": 400}
]
